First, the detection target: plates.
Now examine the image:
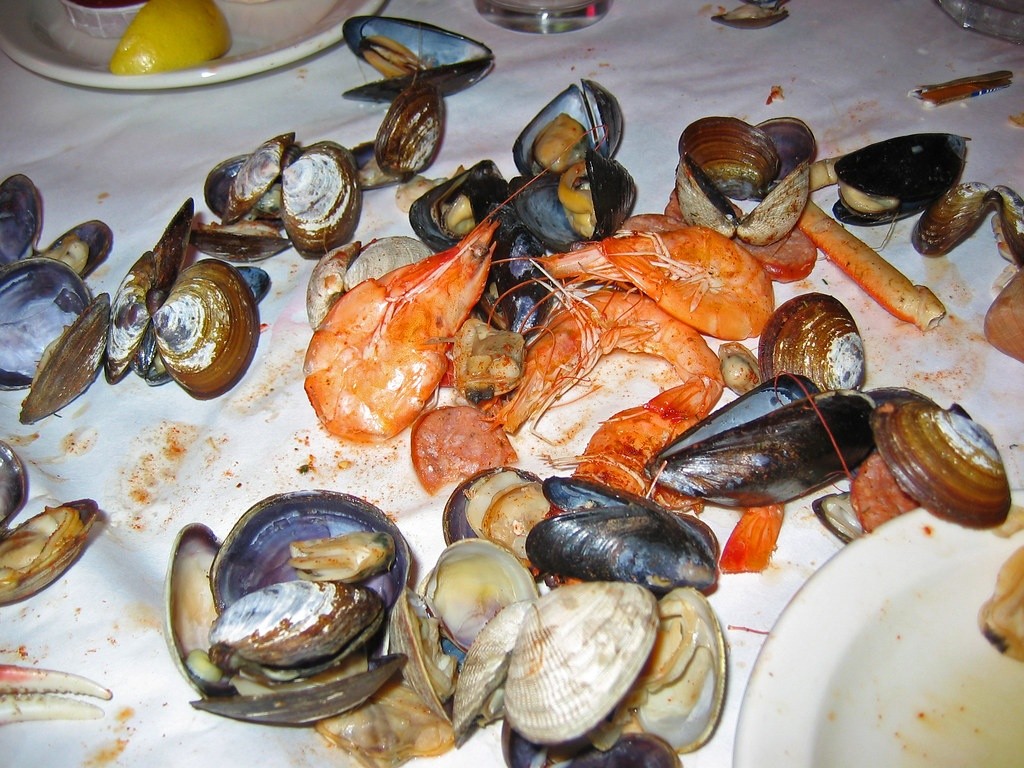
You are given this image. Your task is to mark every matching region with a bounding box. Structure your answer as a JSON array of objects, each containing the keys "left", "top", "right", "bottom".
[
  {"left": 730, "top": 491, "right": 1024, "bottom": 768},
  {"left": 0, "top": 0, "right": 388, "bottom": 92}
]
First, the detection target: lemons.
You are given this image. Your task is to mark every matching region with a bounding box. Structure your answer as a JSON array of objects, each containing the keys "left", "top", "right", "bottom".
[{"left": 109, "top": 0, "right": 232, "bottom": 74}]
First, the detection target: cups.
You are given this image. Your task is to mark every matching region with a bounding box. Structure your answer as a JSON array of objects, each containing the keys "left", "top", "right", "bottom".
[
  {"left": 472, "top": 0, "right": 615, "bottom": 35},
  {"left": 934, "top": 0, "right": 1024, "bottom": 45}
]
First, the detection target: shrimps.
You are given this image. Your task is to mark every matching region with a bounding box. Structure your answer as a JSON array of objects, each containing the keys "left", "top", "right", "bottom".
[{"left": 301, "top": 211, "right": 824, "bottom": 578}]
